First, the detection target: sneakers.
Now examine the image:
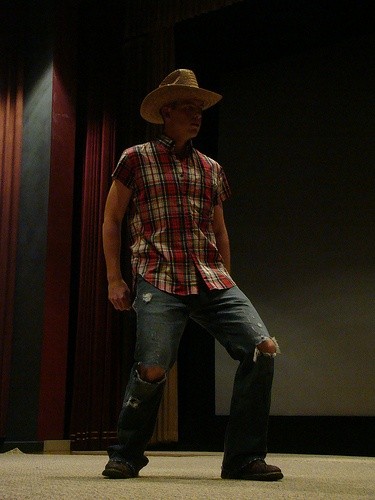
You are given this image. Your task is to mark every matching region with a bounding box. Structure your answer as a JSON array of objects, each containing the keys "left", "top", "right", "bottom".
[
  {"left": 221, "top": 461, "right": 283, "bottom": 480},
  {"left": 102, "top": 461, "right": 138, "bottom": 478}
]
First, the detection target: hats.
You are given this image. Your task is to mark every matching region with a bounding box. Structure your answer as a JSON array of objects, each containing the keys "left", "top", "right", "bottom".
[{"left": 140, "top": 69, "right": 223, "bottom": 124}]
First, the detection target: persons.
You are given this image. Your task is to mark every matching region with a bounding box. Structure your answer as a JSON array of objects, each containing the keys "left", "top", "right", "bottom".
[{"left": 102, "top": 69, "right": 284, "bottom": 481}]
